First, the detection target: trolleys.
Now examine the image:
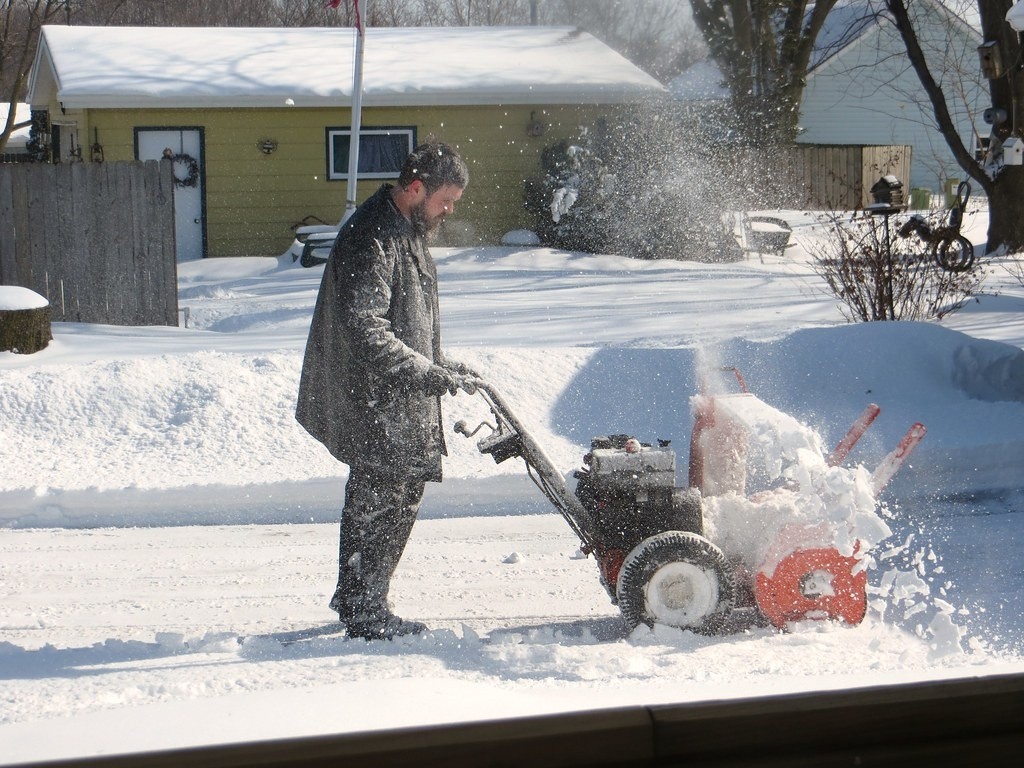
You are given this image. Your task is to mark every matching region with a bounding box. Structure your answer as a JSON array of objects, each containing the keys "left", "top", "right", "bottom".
[
  {"left": 444, "top": 361, "right": 925, "bottom": 637},
  {"left": 741, "top": 214, "right": 799, "bottom": 264}
]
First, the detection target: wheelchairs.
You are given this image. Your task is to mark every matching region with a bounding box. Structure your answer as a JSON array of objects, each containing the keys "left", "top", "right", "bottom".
[{"left": 897, "top": 182, "right": 974, "bottom": 272}]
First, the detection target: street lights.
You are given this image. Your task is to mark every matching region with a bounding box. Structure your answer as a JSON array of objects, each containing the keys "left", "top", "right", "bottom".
[{"left": 863, "top": 175, "right": 907, "bottom": 321}]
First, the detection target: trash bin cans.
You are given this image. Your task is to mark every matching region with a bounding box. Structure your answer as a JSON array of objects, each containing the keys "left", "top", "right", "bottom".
[
  {"left": 911, "top": 187, "right": 931, "bottom": 211},
  {"left": 869, "top": 177, "right": 903, "bottom": 214}
]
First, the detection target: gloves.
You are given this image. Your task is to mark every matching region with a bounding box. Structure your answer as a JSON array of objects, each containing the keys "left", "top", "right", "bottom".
[{"left": 424, "top": 360, "right": 483, "bottom": 397}]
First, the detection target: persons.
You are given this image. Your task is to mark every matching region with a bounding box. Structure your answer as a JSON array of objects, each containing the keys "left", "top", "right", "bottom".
[{"left": 296, "top": 141, "right": 468, "bottom": 640}]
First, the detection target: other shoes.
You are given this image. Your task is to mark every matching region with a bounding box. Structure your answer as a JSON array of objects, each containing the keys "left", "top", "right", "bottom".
[{"left": 345, "top": 610, "right": 427, "bottom": 641}]
